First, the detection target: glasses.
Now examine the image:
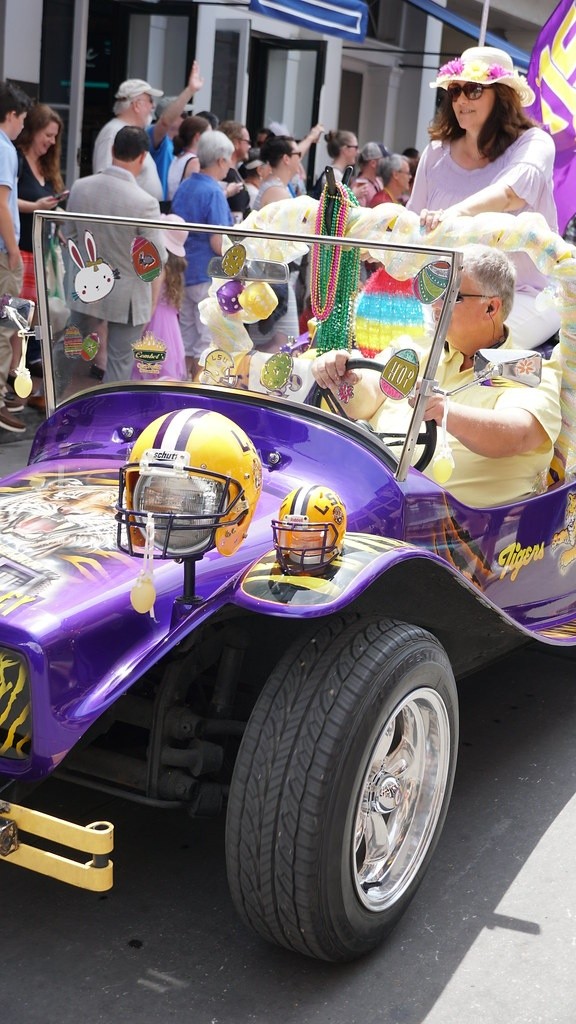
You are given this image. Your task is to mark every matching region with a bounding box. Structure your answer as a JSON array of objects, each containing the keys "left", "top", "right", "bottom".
[
  {"left": 179, "top": 111, "right": 188, "bottom": 119},
  {"left": 235, "top": 138, "right": 252, "bottom": 144},
  {"left": 281, "top": 152, "right": 301, "bottom": 157},
  {"left": 347, "top": 145, "right": 358, "bottom": 149},
  {"left": 441, "top": 291, "right": 495, "bottom": 303},
  {"left": 447, "top": 81, "right": 495, "bottom": 102}
]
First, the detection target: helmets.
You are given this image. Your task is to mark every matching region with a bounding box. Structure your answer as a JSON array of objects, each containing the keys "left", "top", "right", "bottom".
[
  {"left": 272, "top": 485, "right": 348, "bottom": 575},
  {"left": 115, "top": 407, "right": 263, "bottom": 561}
]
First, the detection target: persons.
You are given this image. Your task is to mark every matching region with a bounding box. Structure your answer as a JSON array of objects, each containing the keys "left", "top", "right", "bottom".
[
  {"left": 406, "top": 46, "right": 560, "bottom": 235},
  {"left": 61, "top": 60, "right": 324, "bottom": 402},
  {"left": 0, "top": 80, "right": 69, "bottom": 431},
  {"left": 314, "top": 127, "right": 421, "bottom": 210},
  {"left": 313, "top": 240, "right": 561, "bottom": 508}
]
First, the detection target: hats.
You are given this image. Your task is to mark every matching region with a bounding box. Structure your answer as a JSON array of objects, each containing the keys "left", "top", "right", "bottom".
[
  {"left": 154, "top": 95, "right": 196, "bottom": 118},
  {"left": 114, "top": 79, "right": 164, "bottom": 99},
  {"left": 428, "top": 47, "right": 536, "bottom": 108},
  {"left": 161, "top": 213, "right": 188, "bottom": 258},
  {"left": 357, "top": 142, "right": 392, "bottom": 161}
]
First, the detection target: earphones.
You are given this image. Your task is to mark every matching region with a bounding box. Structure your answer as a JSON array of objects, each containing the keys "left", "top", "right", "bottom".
[{"left": 487, "top": 304, "right": 494, "bottom": 313}]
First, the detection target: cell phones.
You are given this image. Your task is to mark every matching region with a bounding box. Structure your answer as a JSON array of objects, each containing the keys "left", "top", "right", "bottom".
[{"left": 51, "top": 191, "right": 70, "bottom": 201}]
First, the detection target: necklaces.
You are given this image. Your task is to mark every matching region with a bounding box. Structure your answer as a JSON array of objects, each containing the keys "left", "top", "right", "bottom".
[
  {"left": 366, "top": 175, "right": 381, "bottom": 192},
  {"left": 311, "top": 178, "right": 360, "bottom": 356}
]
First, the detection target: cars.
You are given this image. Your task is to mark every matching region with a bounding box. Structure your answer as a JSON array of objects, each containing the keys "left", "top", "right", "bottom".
[{"left": 0, "top": 166, "right": 576, "bottom": 961}]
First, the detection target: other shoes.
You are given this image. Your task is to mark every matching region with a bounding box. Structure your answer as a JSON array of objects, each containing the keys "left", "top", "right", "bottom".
[
  {"left": 0, "top": 412, "right": 27, "bottom": 432},
  {"left": 27, "top": 395, "right": 45, "bottom": 410},
  {"left": 0, "top": 396, "right": 24, "bottom": 411}
]
